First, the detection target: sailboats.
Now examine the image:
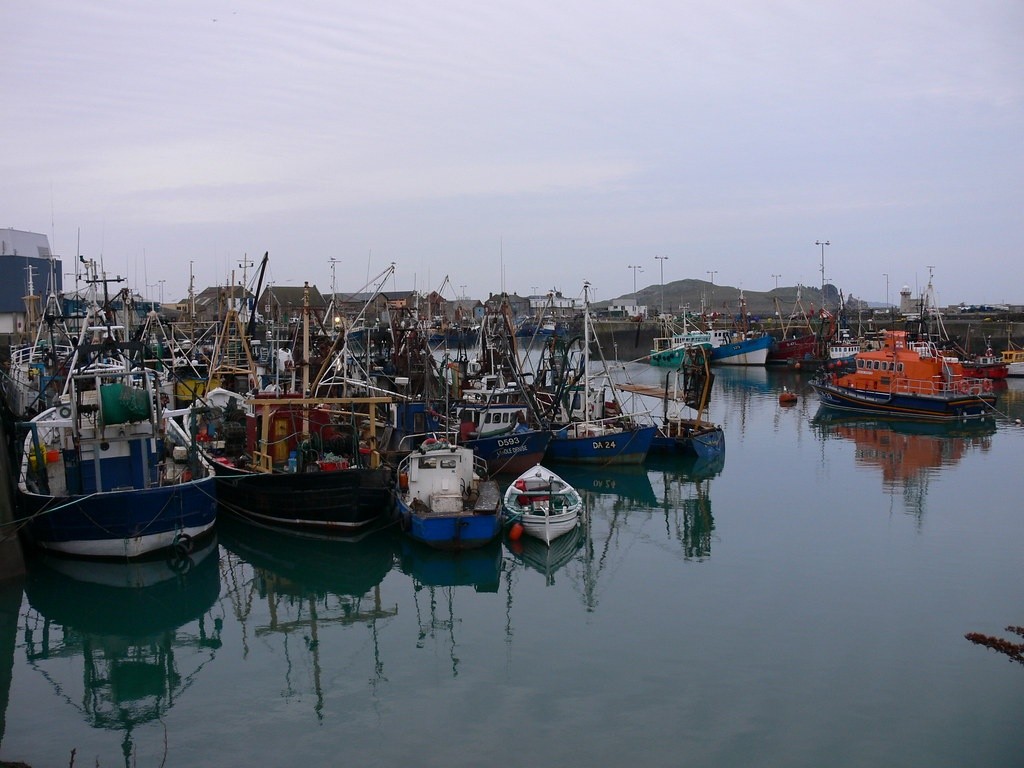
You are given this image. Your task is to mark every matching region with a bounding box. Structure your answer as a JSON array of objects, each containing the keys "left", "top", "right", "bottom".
[
  {"left": 549, "top": 461, "right": 659, "bottom": 614},
  {"left": 216, "top": 524, "right": 396, "bottom": 724},
  {"left": 183, "top": 282, "right": 396, "bottom": 543}
]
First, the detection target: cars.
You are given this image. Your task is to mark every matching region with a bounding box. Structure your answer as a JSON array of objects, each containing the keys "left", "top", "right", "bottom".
[
  {"left": 873, "top": 309, "right": 884, "bottom": 315},
  {"left": 861, "top": 309, "right": 871, "bottom": 314}
]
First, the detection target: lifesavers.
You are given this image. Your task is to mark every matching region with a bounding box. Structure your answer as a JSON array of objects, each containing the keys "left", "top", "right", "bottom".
[
  {"left": 983, "top": 378, "right": 991, "bottom": 392},
  {"left": 422, "top": 438, "right": 450, "bottom": 446},
  {"left": 959, "top": 381, "right": 969, "bottom": 394},
  {"left": 986, "top": 348, "right": 993, "bottom": 357}
]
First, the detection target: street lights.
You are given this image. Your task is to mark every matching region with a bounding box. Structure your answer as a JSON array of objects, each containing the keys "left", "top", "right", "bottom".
[
  {"left": 460, "top": 285, "right": 467, "bottom": 296},
  {"left": 706, "top": 270, "right": 718, "bottom": 289},
  {"left": 628, "top": 265, "right": 642, "bottom": 306},
  {"left": 655, "top": 256, "right": 668, "bottom": 314},
  {"left": 815, "top": 241, "right": 830, "bottom": 309},
  {"left": 883, "top": 273, "right": 888, "bottom": 308},
  {"left": 532, "top": 287, "right": 538, "bottom": 294},
  {"left": 772, "top": 274, "right": 782, "bottom": 296}
]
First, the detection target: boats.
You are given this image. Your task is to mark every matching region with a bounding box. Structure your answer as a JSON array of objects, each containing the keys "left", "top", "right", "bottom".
[
  {"left": 506, "top": 464, "right": 582, "bottom": 546},
  {"left": 2, "top": 251, "right": 726, "bottom": 478},
  {"left": 808, "top": 294, "right": 998, "bottom": 420},
  {"left": 650, "top": 303, "right": 712, "bottom": 367},
  {"left": 23, "top": 536, "right": 223, "bottom": 768},
  {"left": 393, "top": 336, "right": 503, "bottom": 547},
  {"left": 505, "top": 528, "right": 584, "bottom": 585},
  {"left": 960, "top": 335, "right": 1009, "bottom": 378},
  {"left": 710, "top": 287, "right": 773, "bottom": 366},
  {"left": 969, "top": 379, "right": 1008, "bottom": 393},
  {"left": 808, "top": 404, "right": 998, "bottom": 517},
  {"left": 767, "top": 284, "right": 821, "bottom": 361},
  {"left": 16, "top": 254, "right": 219, "bottom": 558},
  {"left": 996, "top": 320, "right": 1024, "bottom": 376},
  {"left": 395, "top": 541, "right": 503, "bottom": 676}
]
